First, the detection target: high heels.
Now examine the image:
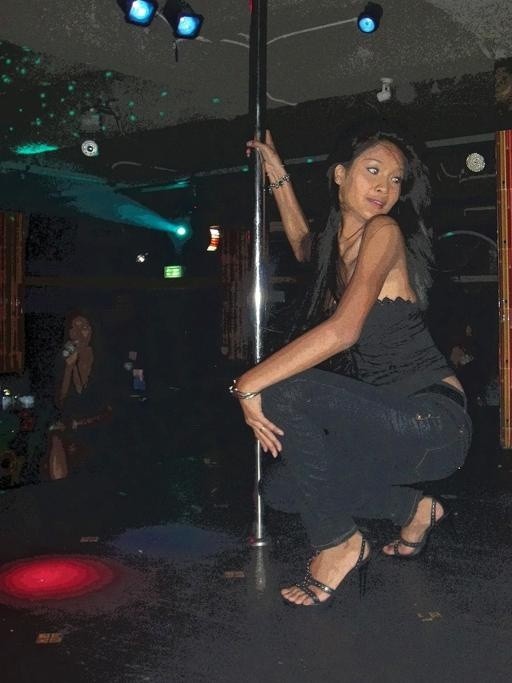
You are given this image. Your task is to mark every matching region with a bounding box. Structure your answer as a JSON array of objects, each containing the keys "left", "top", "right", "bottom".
[
  {"left": 283, "top": 534, "right": 374, "bottom": 604},
  {"left": 382, "top": 500, "right": 452, "bottom": 560}
]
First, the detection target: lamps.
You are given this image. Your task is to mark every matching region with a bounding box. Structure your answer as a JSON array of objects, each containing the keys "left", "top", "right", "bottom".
[
  {"left": 356, "top": 0, "right": 385, "bottom": 35},
  {"left": 116, "top": 0, "right": 205, "bottom": 39}
]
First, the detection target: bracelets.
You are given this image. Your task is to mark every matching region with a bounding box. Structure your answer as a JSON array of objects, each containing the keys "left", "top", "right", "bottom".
[
  {"left": 264, "top": 172, "right": 291, "bottom": 195},
  {"left": 228, "top": 377, "right": 259, "bottom": 403}
]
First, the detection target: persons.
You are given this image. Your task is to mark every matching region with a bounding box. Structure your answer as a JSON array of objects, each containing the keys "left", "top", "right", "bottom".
[
  {"left": 226, "top": 123, "right": 475, "bottom": 608},
  {"left": 34, "top": 309, "right": 123, "bottom": 477}
]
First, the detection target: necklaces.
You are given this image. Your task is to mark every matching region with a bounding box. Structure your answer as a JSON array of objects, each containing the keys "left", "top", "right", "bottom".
[{"left": 337, "top": 223, "right": 365, "bottom": 243}]
